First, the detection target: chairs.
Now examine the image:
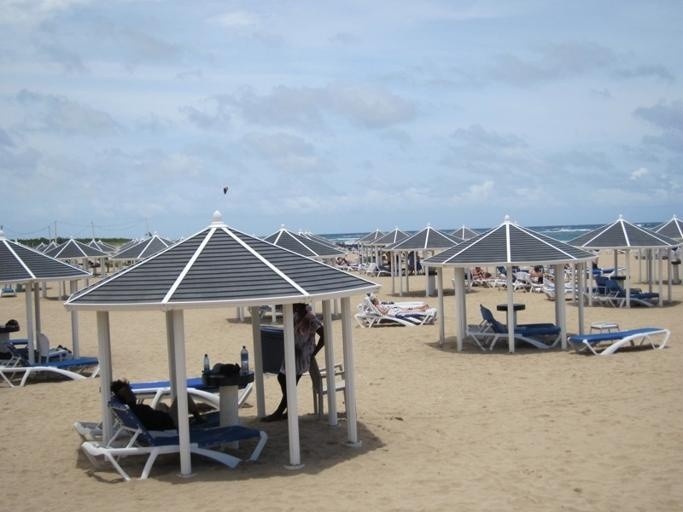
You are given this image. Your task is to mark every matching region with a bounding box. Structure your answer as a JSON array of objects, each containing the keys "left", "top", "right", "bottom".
[
  {"left": 77, "top": 367, "right": 269, "bottom": 484},
  {"left": 330, "top": 252, "right": 436, "bottom": 278},
  {"left": 463, "top": 266, "right": 669, "bottom": 359},
  {"left": 1, "top": 340, "right": 100, "bottom": 389},
  {"left": 309, "top": 356, "right": 349, "bottom": 421},
  {"left": 354, "top": 292, "right": 437, "bottom": 331}
]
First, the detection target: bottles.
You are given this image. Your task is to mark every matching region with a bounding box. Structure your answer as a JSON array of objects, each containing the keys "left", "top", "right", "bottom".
[
  {"left": 203, "top": 353, "right": 209, "bottom": 373},
  {"left": 240, "top": 346, "right": 249, "bottom": 374}
]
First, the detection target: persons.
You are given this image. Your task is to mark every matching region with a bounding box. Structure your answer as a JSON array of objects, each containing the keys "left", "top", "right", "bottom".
[
  {"left": 260, "top": 304, "right": 324, "bottom": 422},
  {"left": 475, "top": 264, "right": 544, "bottom": 285},
  {"left": 0, "top": 319, "right": 29, "bottom": 360},
  {"left": 370, "top": 296, "right": 428, "bottom": 316},
  {"left": 337, "top": 257, "right": 350, "bottom": 266},
  {"left": 111, "top": 379, "right": 207, "bottom": 431},
  {"left": 385, "top": 251, "right": 402, "bottom": 265}
]
